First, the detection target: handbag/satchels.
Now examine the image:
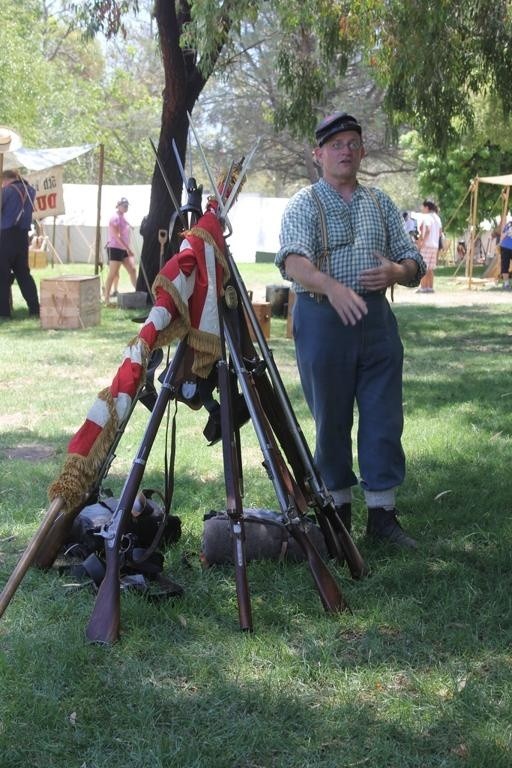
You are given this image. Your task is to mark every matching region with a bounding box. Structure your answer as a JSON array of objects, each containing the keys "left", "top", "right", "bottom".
[
  {"left": 72, "top": 497, "right": 181, "bottom": 554},
  {"left": 201, "top": 510, "right": 329, "bottom": 566}
]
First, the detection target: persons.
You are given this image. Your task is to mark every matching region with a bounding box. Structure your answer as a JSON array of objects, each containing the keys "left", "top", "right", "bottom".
[
  {"left": 397, "top": 199, "right": 512, "bottom": 294},
  {"left": 103, "top": 195, "right": 138, "bottom": 308},
  {"left": 274, "top": 112, "right": 425, "bottom": 565},
  {"left": 0, "top": 169, "right": 42, "bottom": 322}
]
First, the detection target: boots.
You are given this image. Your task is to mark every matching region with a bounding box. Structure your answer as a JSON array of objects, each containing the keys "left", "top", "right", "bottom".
[
  {"left": 321, "top": 504, "right": 352, "bottom": 558},
  {"left": 366, "top": 506, "right": 419, "bottom": 552}
]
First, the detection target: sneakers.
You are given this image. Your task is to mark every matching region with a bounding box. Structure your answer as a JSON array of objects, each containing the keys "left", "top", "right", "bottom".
[{"left": 416, "top": 288, "right": 434, "bottom": 293}]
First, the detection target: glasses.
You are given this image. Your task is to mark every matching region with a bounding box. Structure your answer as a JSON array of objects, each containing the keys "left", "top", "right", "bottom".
[{"left": 330, "top": 142, "right": 360, "bottom": 150}]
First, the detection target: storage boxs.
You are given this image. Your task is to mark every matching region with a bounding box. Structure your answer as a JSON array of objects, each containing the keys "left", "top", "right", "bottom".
[
  {"left": 28, "top": 250, "right": 49, "bottom": 270},
  {"left": 39, "top": 275, "right": 101, "bottom": 330},
  {"left": 242, "top": 302, "right": 272, "bottom": 342},
  {"left": 286, "top": 290, "right": 297, "bottom": 339},
  {"left": 116, "top": 291, "right": 148, "bottom": 310}
]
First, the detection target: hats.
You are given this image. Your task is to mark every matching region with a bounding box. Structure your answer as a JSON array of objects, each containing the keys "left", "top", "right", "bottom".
[
  {"left": 1, "top": 128, "right": 22, "bottom": 156},
  {"left": 314, "top": 112, "right": 361, "bottom": 147}
]
